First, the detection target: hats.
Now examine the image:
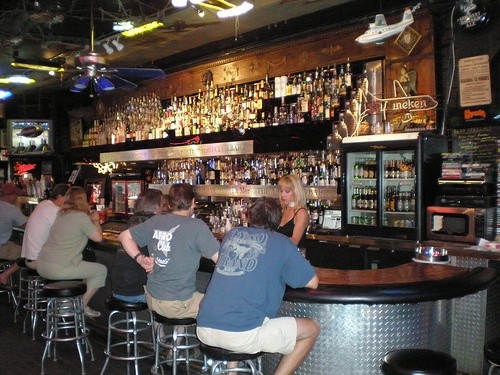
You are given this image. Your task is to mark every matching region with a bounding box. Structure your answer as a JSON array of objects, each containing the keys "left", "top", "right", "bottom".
[{"left": 0, "top": 182, "right": 25, "bottom": 198}]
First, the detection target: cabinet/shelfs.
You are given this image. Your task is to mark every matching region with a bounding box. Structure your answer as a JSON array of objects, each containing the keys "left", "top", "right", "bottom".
[{"left": 76, "top": 61, "right": 368, "bottom": 233}]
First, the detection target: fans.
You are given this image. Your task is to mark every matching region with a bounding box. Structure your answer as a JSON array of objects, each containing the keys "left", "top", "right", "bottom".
[{"left": 49, "top": 0, "right": 167, "bottom": 93}]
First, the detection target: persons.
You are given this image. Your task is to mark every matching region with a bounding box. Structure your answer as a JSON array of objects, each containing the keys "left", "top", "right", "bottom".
[
  {"left": 194, "top": 196, "right": 320, "bottom": 375},
  {"left": 118, "top": 182, "right": 220, "bottom": 368},
  {"left": 20, "top": 183, "right": 71, "bottom": 270},
  {"left": 0, "top": 183, "right": 30, "bottom": 291},
  {"left": 275, "top": 174, "right": 310, "bottom": 258},
  {"left": 110, "top": 188, "right": 164, "bottom": 351},
  {"left": 37, "top": 187, "right": 108, "bottom": 317}
]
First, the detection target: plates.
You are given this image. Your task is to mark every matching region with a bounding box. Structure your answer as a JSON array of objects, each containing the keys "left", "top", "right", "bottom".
[{"left": 413, "top": 246, "right": 448, "bottom": 261}]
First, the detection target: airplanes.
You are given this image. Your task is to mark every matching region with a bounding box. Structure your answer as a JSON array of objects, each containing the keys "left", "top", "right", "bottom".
[{"left": 354, "top": 7, "right": 414, "bottom": 49}]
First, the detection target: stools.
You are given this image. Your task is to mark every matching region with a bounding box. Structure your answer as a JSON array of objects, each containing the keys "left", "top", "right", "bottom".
[
  {"left": 484, "top": 336, "right": 500, "bottom": 375},
  {"left": 100, "top": 293, "right": 164, "bottom": 375},
  {"left": 0, "top": 258, "right": 94, "bottom": 375},
  {"left": 150, "top": 312, "right": 211, "bottom": 375},
  {"left": 200, "top": 342, "right": 266, "bottom": 375},
  {"left": 381, "top": 349, "right": 457, "bottom": 375}
]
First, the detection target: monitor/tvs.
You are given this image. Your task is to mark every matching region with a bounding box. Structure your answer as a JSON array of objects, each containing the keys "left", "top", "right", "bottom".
[{"left": 7, "top": 119, "right": 54, "bottom": 154}]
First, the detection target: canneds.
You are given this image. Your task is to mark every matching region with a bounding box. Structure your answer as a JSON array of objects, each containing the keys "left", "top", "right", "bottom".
[{"left": 351, "top": 215, "right": 415, "bottom": 228}]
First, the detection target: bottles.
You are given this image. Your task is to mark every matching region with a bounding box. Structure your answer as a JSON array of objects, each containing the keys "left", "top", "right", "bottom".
[
  {"left": 352, "top": 159, "right": 416, "bottom": 228},
  {"left": 151, "top": 147, "right": 342, "bottom": 187},
  {"left": 82, "top": 61, "right": 351, "bottom": 144},
  {"left": 209, "top": 199, "right": 324, "bottom": 232}
]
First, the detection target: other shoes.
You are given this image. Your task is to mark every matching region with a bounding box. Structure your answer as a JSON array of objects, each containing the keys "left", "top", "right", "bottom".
[
  {"left": 84, "top": 306, "right": 101, "bottom": 318},
  {"left": 0, "top": 283, "right": 16, "bottom": 291}
]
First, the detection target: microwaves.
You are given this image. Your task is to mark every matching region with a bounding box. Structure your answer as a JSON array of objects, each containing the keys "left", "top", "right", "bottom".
[{"left": 423, "top": 206, "right": 487, "bottom": 243}]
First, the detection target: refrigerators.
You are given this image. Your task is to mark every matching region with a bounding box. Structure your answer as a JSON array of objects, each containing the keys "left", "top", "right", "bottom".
[{"left": 340, "top": 132, "right": 449, "bottom": 241}]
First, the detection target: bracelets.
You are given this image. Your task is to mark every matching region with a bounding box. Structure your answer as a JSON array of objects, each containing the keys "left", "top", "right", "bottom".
[{"left": 133, "top": 253, "right": 142, "bottom": 262}]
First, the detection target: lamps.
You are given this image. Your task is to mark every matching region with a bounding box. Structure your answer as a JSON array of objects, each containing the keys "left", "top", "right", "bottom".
[
  {"left": 102, "top": 39, "right": 113, "bottom": 55},
  {"left": 121, "top": 17, "right": 164, "bottom": 37},
  {"left": 112, "top": 34, "right": 124, "bottom": 52},
  {"left": 10, "top": 51, "right": 65, "bottom": 72},
  {"left": 70, "top": 74, "right": 114, "bottom": 99}
]
